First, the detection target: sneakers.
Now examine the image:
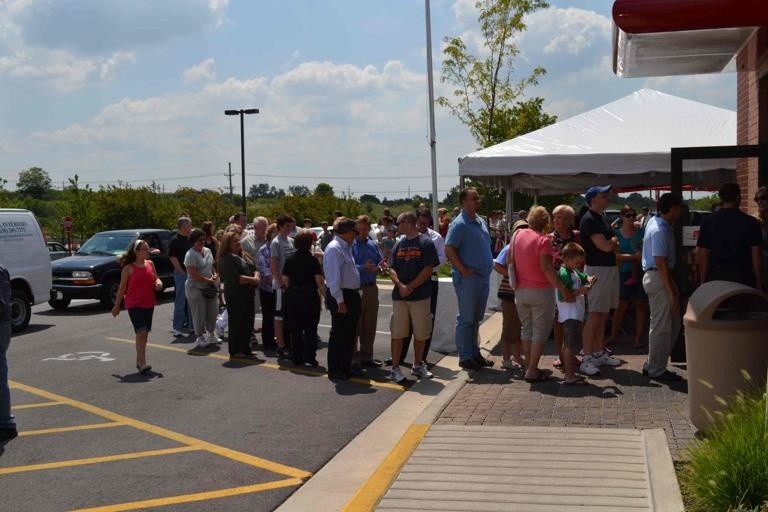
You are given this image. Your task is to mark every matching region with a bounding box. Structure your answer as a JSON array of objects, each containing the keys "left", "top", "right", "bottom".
[
  {"left": 174, "top": 321, "right": 434, "bottom": 383},
  {"left": 0, "top": 428, "right": 18, "bottom": 441}
]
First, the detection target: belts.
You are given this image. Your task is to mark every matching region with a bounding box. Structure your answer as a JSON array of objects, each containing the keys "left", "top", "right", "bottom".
[
  {"left": 362, "top": 282, "right": 376, "bottom": 287},
  {"left": 644, "top": 267, "right": 660, "bottom": 271}
]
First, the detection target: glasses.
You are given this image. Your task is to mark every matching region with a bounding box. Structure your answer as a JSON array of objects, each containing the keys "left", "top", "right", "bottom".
[
  {"left": 624, "top": 213, "right": 636, "bottom": 218},
  {"left": 754, "top": 195, "right": 767, "bottom": 202},
  {"left": 394, "top": 220, "right": 405, "bottom": 226},
  {"left": 352, "top": 229, "right": 360, "bottom": 235}
]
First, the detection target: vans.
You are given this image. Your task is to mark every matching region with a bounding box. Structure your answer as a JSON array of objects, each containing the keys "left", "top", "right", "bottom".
[{"left": 0, "top": 210, "right": 51, "bottom": 327}]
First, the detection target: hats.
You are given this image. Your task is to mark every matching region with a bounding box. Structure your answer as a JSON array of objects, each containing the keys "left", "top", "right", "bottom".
[
  {"left": 512, "top": 220, "right": 529, "bottom": 232},
  {"left": 586, "top": 185, "right": 612, "bottom": 202}
]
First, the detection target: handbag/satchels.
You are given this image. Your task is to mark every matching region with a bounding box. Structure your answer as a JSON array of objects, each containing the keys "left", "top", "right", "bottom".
[
  {"left": 507, "top": 260, "right": 516, "bottom": 290},
  {"left": 201, "top": 285, "right": 217, "bottom": 299}
]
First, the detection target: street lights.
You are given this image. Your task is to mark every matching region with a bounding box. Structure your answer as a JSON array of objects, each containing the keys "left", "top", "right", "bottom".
[{"left": 220, "top": 107, "right": 263, "bottom": 225}]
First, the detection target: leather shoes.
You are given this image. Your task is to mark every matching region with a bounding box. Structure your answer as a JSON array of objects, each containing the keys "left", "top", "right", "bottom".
[
  {"left": 139, "top": 365, "right": 152, "bottom": 374},
  {"left": 650, "top": 370, "right": 682, "bottom": 381},
  {"left": 459, "top": 359, "right": 481, "bottom": 370},
  {"left": 643, "top": 369, "right": 676, "bottom": 378},
  {"left": 474, "top": 352, "right": 494, "bottom": 366}
]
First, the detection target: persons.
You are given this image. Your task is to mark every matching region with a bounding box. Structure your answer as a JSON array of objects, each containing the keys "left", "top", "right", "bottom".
[
  {"left": 169, "top": 202, "right": 460, "bottom": 384},
  {"left": 445, "top": 180, "right": 767, "bottom": 384},
  {"left": 110, "top": 239, "right": 164, "bottom": 376}
]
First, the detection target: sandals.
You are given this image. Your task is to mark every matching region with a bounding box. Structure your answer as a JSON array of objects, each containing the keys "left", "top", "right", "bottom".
[{"left": 501, "top": 345, "right": 620, "bottom": 385}]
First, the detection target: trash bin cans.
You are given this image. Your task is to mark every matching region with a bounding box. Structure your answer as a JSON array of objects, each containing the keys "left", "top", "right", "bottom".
[{"left": 683, "top": 280, "right": 768, "bottom": 433}]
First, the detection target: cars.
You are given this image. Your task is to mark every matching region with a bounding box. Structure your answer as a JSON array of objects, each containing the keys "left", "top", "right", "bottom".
[
  {"left": 51, "top": 226, "right": 184, "bottom": 308},
  {"left": 46, "top": 240, "right": 73, "bottom": 260}
]
